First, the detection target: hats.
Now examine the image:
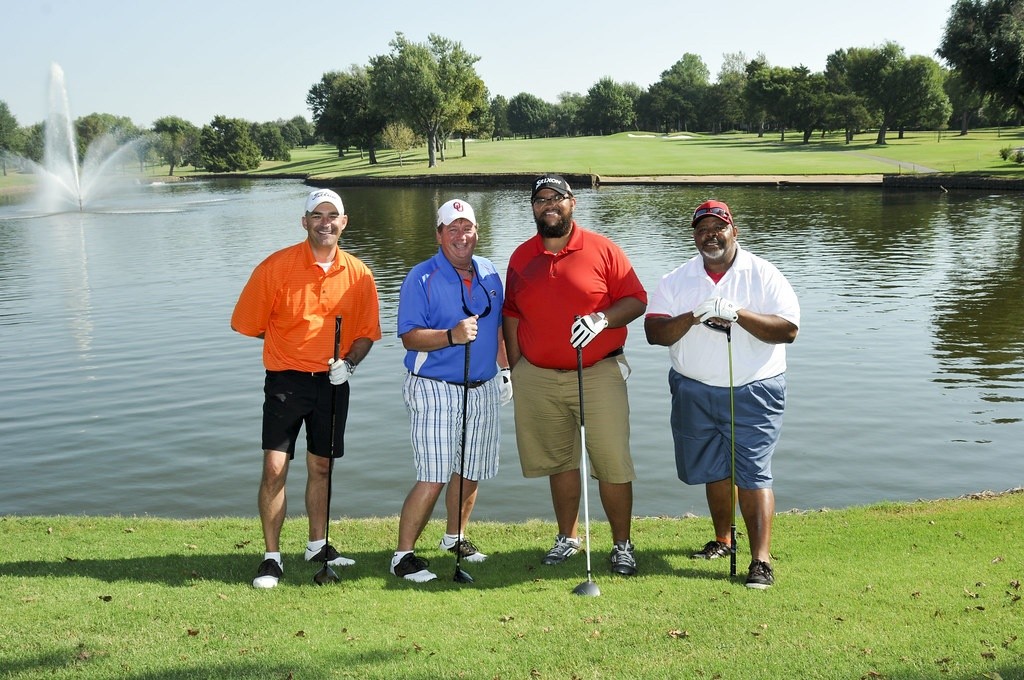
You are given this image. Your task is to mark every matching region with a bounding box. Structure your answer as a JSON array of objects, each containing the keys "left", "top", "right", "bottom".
[
  {"left": 436, "top": 198, "right": 478, "bottom": 228},
  {"left": 532, "top": 172, "right": 574, "bottom": 199},
  {"left": 692, "top": 200, "right": 733, "bottom": 228},
  {"left": 305, "top": 188, "right": 345, "bottom": 217}
]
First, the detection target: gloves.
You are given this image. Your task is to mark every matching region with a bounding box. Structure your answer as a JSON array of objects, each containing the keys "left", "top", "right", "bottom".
[
  {"left": 692, "top": 296, "right": 742, "bottom": 323},
  {"left": 570, "top": 311, "right": 608, "bottom": 349},
  {"left": 499, "top": 370, "right": 513, "bottom": 406},
  {"left": 327, "top": 358, "right": 352, "bottom": 385}
]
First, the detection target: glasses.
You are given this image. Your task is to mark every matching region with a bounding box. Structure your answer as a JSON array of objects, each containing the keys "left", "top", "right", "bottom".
[
  {"left": 462, "top": 283, "right": 492, "bottom": 318},
  {"left": 693, "top": 207, "right": 730, "bottom": 219},
  {"left": 532, "top": 195, "right": 570, "bottom": 205}
]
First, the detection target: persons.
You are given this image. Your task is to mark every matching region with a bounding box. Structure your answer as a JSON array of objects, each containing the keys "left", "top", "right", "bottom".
[
  {"left": 389, "top": 199, "right": 513, "bottom": 584},
  {"left": 643, "top": 201, "right": 801, "bottom": 590},
  {"left": 231, "top": 188, "right": 379, "bottom": 588},
  {"left": 502, "top": 174, "right": 648, "bottom": 578}
]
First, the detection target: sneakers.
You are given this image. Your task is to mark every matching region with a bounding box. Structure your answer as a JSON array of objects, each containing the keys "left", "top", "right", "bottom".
[
  {"left": 389, "top": 553, "right": 437, "bottom": 583},
  {"left": 610, "top": 539, "right": 638, "bottom": 576},
  {"left": 744, "top": 559, "right": 776, "bottom": 589},
  {"left": 252, "top": 558, "right": 284, "bottom": 589},
  {"left": 542, "top": 534, "right": 581, "bottom": 565},
  {"left": 689, "top": 540, "right": 731, "bottom": 560},
  {"left": 438, "top": 538, "right": 488, "bottom": 563},
  {"left": 304, "top": 544, "right": 356, "bottom": 567}
]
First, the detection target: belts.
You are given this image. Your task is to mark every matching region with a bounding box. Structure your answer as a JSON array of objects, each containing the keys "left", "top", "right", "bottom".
[
  {"left": 602, "top": 346, "right": 624, "bottom": 360},
  {"left": 408, "top": 370, "right": 488, "bottom": 388}
]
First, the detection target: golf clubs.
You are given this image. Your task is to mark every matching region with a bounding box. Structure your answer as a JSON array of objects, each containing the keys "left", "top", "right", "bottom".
[
  {"left": 312, "top": 313, "right": 344, "bottom": 587},
  {"left": 567, "top": 312, "right": 604, "bottom": 599},
  {"left": 698, "top": 316, "right": 741, "bottom": 582},
  {"left": 453, "top": 341, "right": 477, "bottom": 586}
]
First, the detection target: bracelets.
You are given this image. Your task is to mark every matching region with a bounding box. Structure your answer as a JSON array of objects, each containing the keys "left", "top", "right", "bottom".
[{"left": 447, "top": 329, "right": 454, "bottom": 347}]
